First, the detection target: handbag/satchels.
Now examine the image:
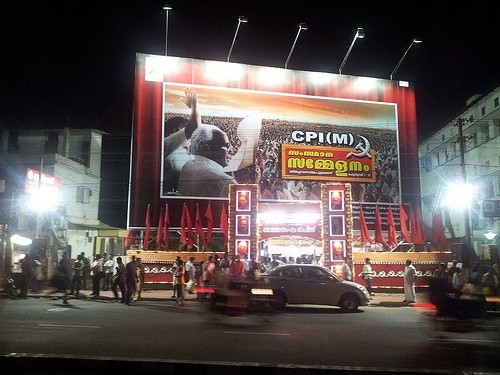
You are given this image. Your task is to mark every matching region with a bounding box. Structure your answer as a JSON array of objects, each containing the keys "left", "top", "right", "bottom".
[
  {"left": 184, "top": 270, "right": 190, "bottom": 283},
  {"left": 358, "top": 272, "right": 364, "bottom": 279}
]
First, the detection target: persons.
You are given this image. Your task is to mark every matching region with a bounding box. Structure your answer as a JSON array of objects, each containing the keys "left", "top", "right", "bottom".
[
  {"left": 361, "top": 258, "right": 374, "bottom": 296},
  {"left": 164, "top": 86, "right": 400, "bottom": 203},
  {"left": 403, "top": 260, "right": 418, "bottom": 303},
  {"left": 432, "top": 260, "right": 500, "bottom": 293},
  {"left": 342, "top": 257, "right": 353, "bottom": 281},
  {"left": 172, "top": 252, "right": 324, "bottom": 305},
  {"left": 20, "top": 251, "right": 145, "bottom": 304}
]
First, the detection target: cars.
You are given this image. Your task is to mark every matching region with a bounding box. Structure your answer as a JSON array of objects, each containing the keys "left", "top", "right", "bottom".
[{"left": 259, "top": 264, "right": 371, "bottom": 311}]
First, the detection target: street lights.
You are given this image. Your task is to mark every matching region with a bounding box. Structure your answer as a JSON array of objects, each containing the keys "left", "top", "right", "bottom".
[
  {"left": 450, "top": 182, "right": 477, "bottom": 272},
  {"left": 32, "top": 184, "right": 60, "bottom": 256},
  {"left": 483, "top": 228, "right": 498, "bottom": 257}
]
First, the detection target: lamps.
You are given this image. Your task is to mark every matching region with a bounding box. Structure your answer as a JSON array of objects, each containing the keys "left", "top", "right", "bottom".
[
  {"left": 163, "top": 5, "right": 172, "bottom": 56},
  {"left": 339, "top": 28, "right": 364, "bottom": 74},
  {"left": 285, "top": 23, "right": 307, "bottom": 69},
  {"left": 390, "top": 38, "right": 422, "bottom": 80},
  {"left": 227, "top": 16, "right": 247, "bottom": 62}
]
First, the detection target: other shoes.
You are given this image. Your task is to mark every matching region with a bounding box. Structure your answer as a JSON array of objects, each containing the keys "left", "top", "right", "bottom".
[
  {"left": 185, "top": 289, "right": 188, "bottom": 292},
  {"left": 133, "top": 297, "right": 141, "bottom": 301},
  {"left": 67, "top": 292, "right": 74, "bottom": 295},
  {"left": 403, "top": 300, "right": 408, "bottom": 302},
  {"left": 189, "top": 292, "right": 194, "bottom": 294},
  {"left": 90, "top": 293, "right": 96, "bottom": 295},
  {"left": 126, "top": 302, "right": 132, "bottom": 306},
  {"left": 178, "top": 299, "right": 184, "bottom": 302},
  {"left": 63, "top": 297, "right": 70, "bottom": 300},
  {"left": 408, "top": 302, "right": 415, "bottom": 303},
  {"left": 172, "top": 296, "right": 176, "bottom": 297},
  {"left": 93, "top": 295, "right": 99, "bottom": 299},
  {"left": 370, "top": 293, "right": 374, "bottom": 296},
  {"left": 112, "top": 297, "right": 119, "bottom": 300},
  {"left": 63, "top": 301, "right": 69, "bottom": 305},
  {"left": 74, "top": 293, "right": 79, "bottom": 296},
  {"left": 119, "top": 299, "right": 127, "bottom": 303},
  {"left": 103, "top": 288, "right": 110, "bottom": 291}
]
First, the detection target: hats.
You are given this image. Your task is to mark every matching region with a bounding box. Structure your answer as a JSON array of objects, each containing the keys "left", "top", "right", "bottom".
[
  {"left": 176, "top": 259, "right": 183, "bottom": 265},
  {"left": 364, "top": 258, "right": 370, "bottom": 263}
]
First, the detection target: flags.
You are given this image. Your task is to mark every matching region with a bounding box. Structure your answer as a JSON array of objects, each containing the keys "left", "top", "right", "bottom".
[
  {"left": 220, "top": 206, "right": 228, "bottom": 244},
  {"left": 205, "top": 202, "right": 214, "bottom": 242},
  {"left": 386, "top": 207, "right": 398, "bottom": 248},
  {"left": 185, "top": 207, "right": 195, "bottom": 248},
  {"left": 360, "top": 207, "right": 375, "bottom": 244},
  {"left": 375, "top": 208, "right": 386, "bottom": 245},
  {"left": 398, "top": 205, "right": 411, "bottom": 243},
  {"left": 195, "top": 205, "right": 205, "bottom": 245},
  {"left": 430, "top": 208, "right": 448, "bottom": 247},
  {"left": 144, "top": 208, "right": 150, "bottom": 248},
  {"left": 409, "top": 206, "right": 425, "bottom": 243},
  {"left": 181, "top": 207, "right": 187, "bottom": 244},
  {"left": 157, "top": 213, "right": 163, "bottom": 249},
  {"left": 164, "top": 208, "right": 170, "bottom": 245}
]
